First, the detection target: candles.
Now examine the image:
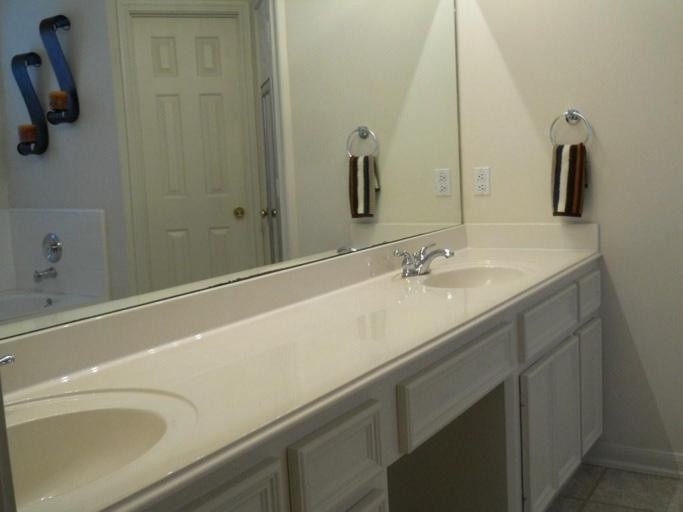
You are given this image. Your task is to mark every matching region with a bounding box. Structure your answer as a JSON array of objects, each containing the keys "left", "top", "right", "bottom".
[
  {"left": 48, "top": 91, "right": 68, "bottom": 109},
  {"left": 18, "top": 125, "right": 38, "bottom": 141}
]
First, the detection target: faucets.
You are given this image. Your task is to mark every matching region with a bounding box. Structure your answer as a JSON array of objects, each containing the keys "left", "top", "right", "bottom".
[
  {"left": 336, "top": 243, "right": 357, "bottom": 253},
  {"left": 32, "top": 265, "right": 57, "bottom": 281},
  {"left": 394, "top": 240, "right": 455, "bottom": 277}
]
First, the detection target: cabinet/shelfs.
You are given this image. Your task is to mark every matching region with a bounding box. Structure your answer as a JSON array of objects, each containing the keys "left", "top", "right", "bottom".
[
  {"left": 390, "top": 263, "right": 602, "bottom": 512},
  {"left": 175, "top": 396, "right": 390, "bottom": 512}
]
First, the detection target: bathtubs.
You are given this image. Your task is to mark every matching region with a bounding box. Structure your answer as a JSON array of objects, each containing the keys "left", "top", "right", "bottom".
[{"left": 0, "top": 289, "right": 101, "bottom": 324}]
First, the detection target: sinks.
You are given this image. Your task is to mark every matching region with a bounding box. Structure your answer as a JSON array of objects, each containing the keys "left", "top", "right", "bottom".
[
  {"left": 420, "top": 265, "right": 530, "bottom": 292},
  {"left": 4, "top": 387, "right": 198, "bottom": 511}
]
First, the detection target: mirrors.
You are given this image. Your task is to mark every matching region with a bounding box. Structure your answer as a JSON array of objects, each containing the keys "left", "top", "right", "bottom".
[{"left": 0, "top": 1, "right": 464, "bottom": 347}]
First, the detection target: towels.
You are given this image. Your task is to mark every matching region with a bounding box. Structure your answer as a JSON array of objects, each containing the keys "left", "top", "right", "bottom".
[
  {"left": 550, "top": 143, "right": 587, "bottom": 216},
  {"left": 348, "top": 155, "right": 380, "bottom": 218}
]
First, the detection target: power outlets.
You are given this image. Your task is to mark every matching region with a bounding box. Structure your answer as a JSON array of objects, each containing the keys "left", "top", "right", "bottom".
[
  {"left": 473, "top": 167, "right": 490, "bottom": 195},
  {"left": 435, "top": 168, "right": 449, "bottom": 197}
]
